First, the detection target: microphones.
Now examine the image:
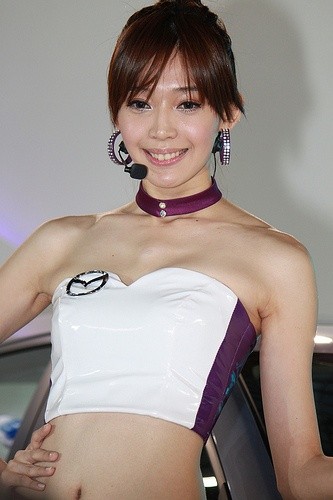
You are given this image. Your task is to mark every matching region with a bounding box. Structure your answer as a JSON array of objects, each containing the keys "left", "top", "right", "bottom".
[{"left": 118, "top": 141, "right": 148, "bottom": 180}]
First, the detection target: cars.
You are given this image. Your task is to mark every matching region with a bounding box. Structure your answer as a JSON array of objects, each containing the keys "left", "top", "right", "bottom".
[{"left": 1, "top": 323, "right": 332, "bottom": 500}]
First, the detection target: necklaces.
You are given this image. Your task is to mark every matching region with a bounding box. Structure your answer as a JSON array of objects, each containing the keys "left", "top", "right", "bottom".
[{"left": 136, "top": 176, "right": 222, "bottom": 218}]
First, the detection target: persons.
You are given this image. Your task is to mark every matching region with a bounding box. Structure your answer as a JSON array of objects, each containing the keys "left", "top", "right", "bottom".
[{"left": 0, "top": 0, "right": 333, "bottom": 500}]
